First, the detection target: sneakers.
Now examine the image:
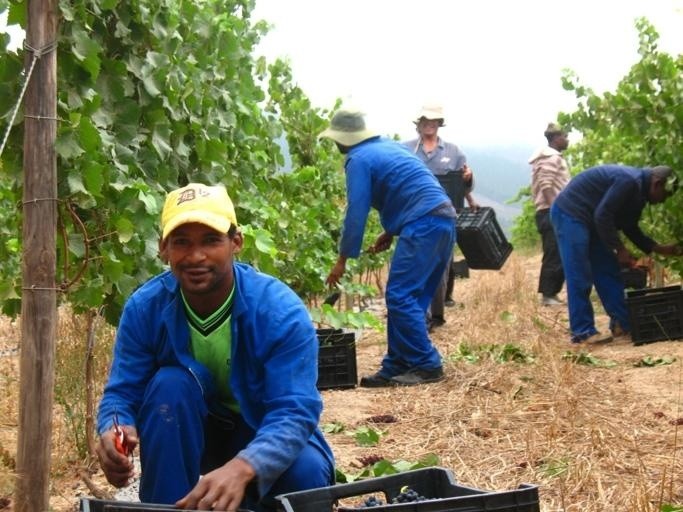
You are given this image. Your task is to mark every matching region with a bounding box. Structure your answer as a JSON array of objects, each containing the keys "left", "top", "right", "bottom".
[
  {"left": 387, "top": 364, "right": 448, "bottom": 388},
  {"left": 430, "top": 317, "right": 445, "bottom": 328},
  {"left": 360, "top": 364, "right": 413, "bottom": 389},
  {"left": 539, "top": 293, "right": 571, "bottom": 307},
  {"left": 444, "top": 296, "right": 455, "bottom": 306},
  {"left": 576, "top": 331, "right": 614, "bottom": 346},
  {"left": 609, "top": 318, "right": 627, "bottom": 338}
]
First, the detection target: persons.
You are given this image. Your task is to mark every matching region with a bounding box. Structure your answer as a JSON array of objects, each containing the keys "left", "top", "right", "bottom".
[
  {"left": 400, "top": 99, "right": 473, "bottom": 328},
  {"left": 443, "top": 191, "right": 479, "bottom": 306},
  {"left": 318, "top": 108, "right": 457, "bottom": 386},
  {"left": 549, "top": 164, "right": 683, "bottom": 342},
  {"left": 529, "top": 123, "right": 572, "bottom": 304},
  {"left": 96, "top": 184, "right": 336, "bottom": 512}
]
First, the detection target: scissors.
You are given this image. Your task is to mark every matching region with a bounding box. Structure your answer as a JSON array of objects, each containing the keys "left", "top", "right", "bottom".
[
  {"left": 359, "top": 243, "right": 383, "bottom": 262},
  {"left": 112, "top": 405, "right": 134, "bottom": 468}
]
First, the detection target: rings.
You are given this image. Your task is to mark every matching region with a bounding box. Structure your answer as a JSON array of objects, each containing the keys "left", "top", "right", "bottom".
[{"left": 200, "top": 497, "right": 209, "bottom": 505}]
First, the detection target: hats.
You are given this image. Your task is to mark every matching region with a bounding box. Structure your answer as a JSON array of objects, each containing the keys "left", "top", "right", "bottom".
[
  {"left": 410, "top": 104, "right": 446, "bottom": 127},
  {"left": 317, "top": 108, "right": 382, "bottom": 148},
  {"left": 160, "top": 182, "right": 239, "bottom": 242}
]
